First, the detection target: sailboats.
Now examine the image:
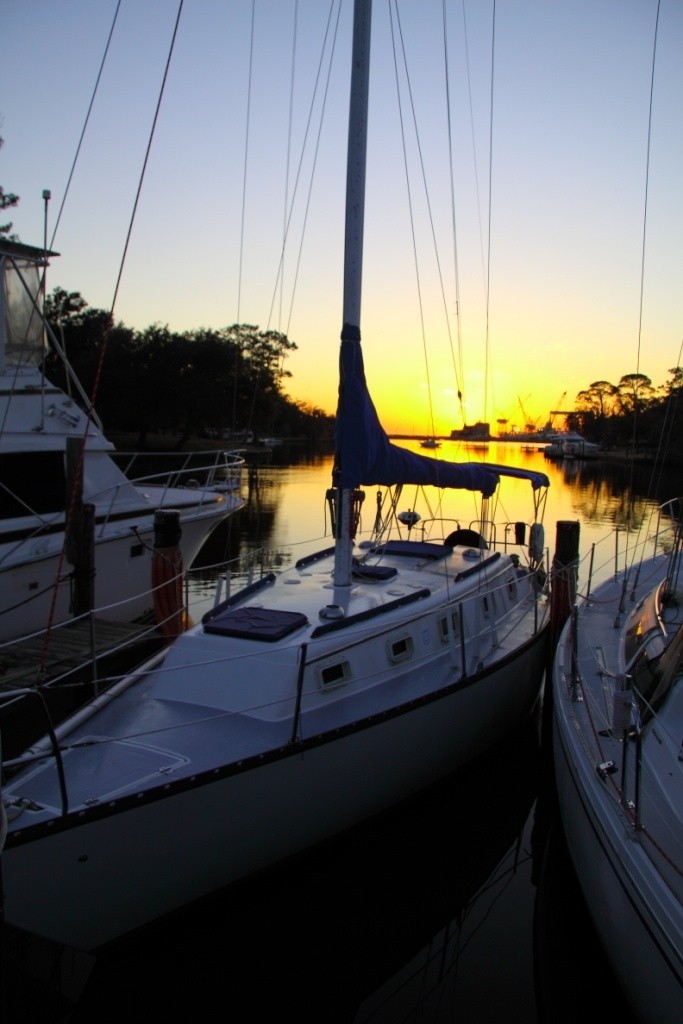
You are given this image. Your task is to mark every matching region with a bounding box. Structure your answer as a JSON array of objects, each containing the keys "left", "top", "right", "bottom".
[{"left": 1, "top": 0, "right": 562, "bottom": 960}]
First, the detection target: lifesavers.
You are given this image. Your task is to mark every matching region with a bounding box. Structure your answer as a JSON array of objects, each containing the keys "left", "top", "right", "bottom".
[{"left": 443, "top": 528, "right": 488, "bottom": 549}]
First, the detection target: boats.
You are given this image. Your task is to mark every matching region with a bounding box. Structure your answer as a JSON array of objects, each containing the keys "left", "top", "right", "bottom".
[
  {"left": 0, "top": 235, "right": 252, "bottom": 654},
  {"left": 544, "top": 432, "right": 602, "bottom": 455},
  {"left": 421, "top": 441, "right": 441, "bottom": 447},
  {"left": 551, "top": 495, "right": 682, "bottom": 1024}
]
